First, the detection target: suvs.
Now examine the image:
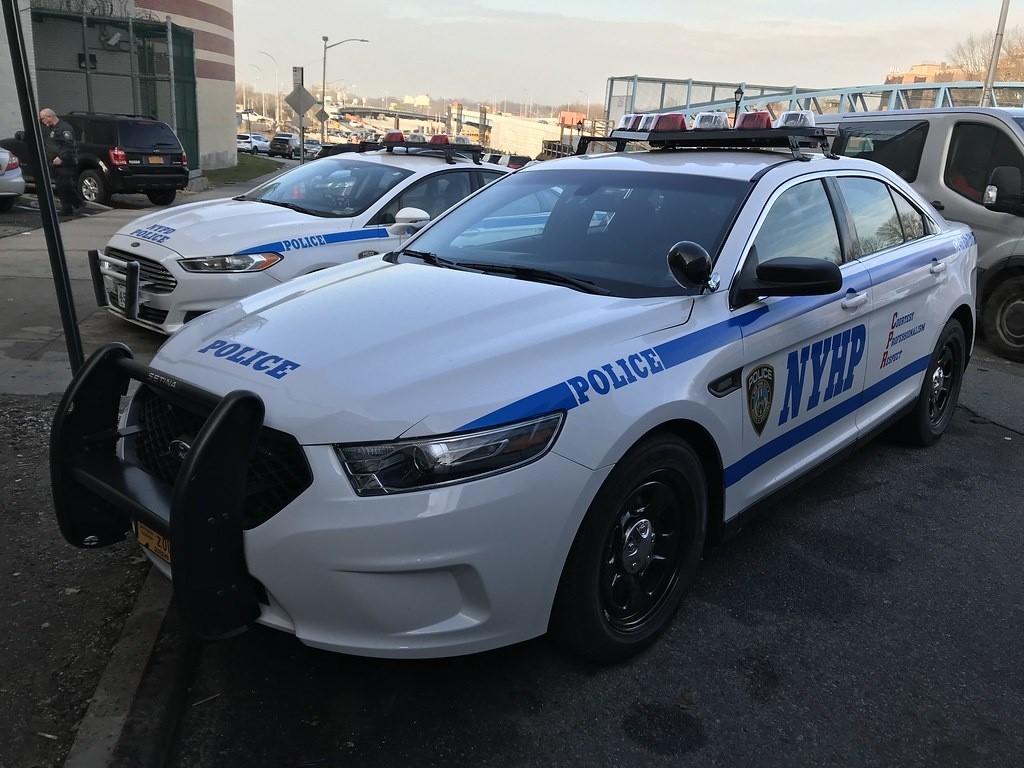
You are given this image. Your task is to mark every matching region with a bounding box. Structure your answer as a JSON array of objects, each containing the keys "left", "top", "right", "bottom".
[{"left": 0, "top": 110, "right": 192, "bottom": 204}]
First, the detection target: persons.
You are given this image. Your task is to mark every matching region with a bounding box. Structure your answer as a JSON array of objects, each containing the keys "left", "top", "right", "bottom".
[
  {"left": 577, "top": 121, "right": 582, "bottom": 135},
  {"left": 334, "top": 120, "right": 340, "bottom": 128},
  {"left": 40, "top": 108, "right": 86, "bottom": 216}
]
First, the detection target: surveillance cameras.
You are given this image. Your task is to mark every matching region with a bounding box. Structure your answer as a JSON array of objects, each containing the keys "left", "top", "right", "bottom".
[{"left": 107, "top": 32, "right": 121, "bottom": 46}]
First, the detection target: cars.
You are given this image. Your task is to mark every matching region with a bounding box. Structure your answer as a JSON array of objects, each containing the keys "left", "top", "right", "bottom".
[
  {"left": 237, "top": 132, "right": 477, "bottom": 160},
  {"left": 87, "top": 131, "right": 531, "bottom": 334},
  {"left": 799, "top": 107, "right": 1024, "bottom": 361},
  {"left": 49, "top": 113, "right": 979, "bottom": 671}
]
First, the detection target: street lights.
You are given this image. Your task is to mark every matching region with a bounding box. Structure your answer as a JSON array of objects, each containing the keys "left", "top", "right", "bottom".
[{"left": 321, "top": 35, "right": 369, "bottom": 143}]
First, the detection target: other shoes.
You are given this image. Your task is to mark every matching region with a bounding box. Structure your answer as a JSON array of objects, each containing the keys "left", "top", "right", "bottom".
[
  {"left": 58, "top": 205, "right": 73, "bottom": 215},
  {"left": 74, "top": 202, "right": 87, "bottom": 214}
]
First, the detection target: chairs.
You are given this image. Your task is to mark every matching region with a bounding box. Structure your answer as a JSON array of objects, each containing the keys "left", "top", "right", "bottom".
[
  {"left": 362, "top": 179, "right": 407, "bottom": 216},
  {"left": 966, "top": 141, "right": 1004, "bottom": 197},
  {"left": 593, "top": 198, "right": 667, "bottom": 264},
  {"left": 430, "top": 178, "right": 465, "bottom": 220}
]
